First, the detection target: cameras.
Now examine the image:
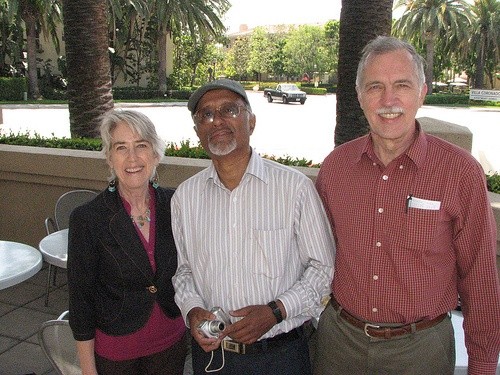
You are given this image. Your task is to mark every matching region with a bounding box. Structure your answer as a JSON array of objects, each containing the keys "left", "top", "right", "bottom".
[{"left": 197, "top": 307, "right": 232, "bottom": 346}]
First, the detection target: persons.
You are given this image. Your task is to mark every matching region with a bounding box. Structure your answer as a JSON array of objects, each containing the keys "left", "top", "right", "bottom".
[
  {"left": 170, "top": 79, "right": 335, "bottom": 375},
  {"left": 313, "top": 37, "right": 500, "bottom": 375},
  {"left": 68, "top": 111, "right": 187, "bottom": 375}
]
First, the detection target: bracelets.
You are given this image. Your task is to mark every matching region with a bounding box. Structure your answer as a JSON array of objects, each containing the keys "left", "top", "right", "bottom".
[{"left": 267, "top": 301, "right": 283, "bottom": 324}]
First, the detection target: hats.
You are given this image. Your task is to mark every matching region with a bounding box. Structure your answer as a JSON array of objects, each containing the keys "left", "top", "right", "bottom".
[{"left": 187, "top": 80, "right": 250, "bottom": 116}]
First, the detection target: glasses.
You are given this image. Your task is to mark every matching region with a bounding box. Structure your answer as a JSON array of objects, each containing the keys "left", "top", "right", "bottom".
[{"left": 192, "top": 104, "right": 251, "bottom": 125}]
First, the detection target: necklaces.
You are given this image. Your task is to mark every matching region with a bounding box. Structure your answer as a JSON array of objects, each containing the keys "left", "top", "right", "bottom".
[{"left": 131, "top": 208, "right": 151, "bottom": 226}]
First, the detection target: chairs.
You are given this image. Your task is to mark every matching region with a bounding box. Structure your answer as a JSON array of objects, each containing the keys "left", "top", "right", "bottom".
[
  {"left": 45, "top": 188, "right": 100, "bottom": 307},
  {"left": 38, "top": 311, "right": 84, "bottom": 375}
]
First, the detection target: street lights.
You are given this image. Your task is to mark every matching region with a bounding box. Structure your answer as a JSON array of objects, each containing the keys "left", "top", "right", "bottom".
[{"left": 212, "top": 58, "right": 217, "bottom": 79}]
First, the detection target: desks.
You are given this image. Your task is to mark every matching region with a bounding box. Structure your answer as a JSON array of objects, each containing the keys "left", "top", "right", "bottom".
[
  {"left": 1, "top": 239, "right": 43, "bottom": 291},
  {"left": 39, "top": 228, "right": 71, "bottom": 269}
]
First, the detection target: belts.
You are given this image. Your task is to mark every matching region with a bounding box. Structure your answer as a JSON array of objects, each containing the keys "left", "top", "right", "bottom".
[
  {"left": 329, "top": 294, "right": 447, "bottom": 338},
  {"left": 221, "top": 329, "right": 302, "bottom": 354}
]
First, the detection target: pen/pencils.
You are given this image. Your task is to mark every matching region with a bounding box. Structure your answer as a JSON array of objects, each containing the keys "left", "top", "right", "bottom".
[{"left": 406, "top": 195, "right": 412, "bottom": 214}]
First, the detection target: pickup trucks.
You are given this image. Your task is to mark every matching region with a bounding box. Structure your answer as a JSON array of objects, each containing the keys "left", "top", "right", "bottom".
[{"left": 264, "top": 83, "right": 307, "bottom": 105}]
